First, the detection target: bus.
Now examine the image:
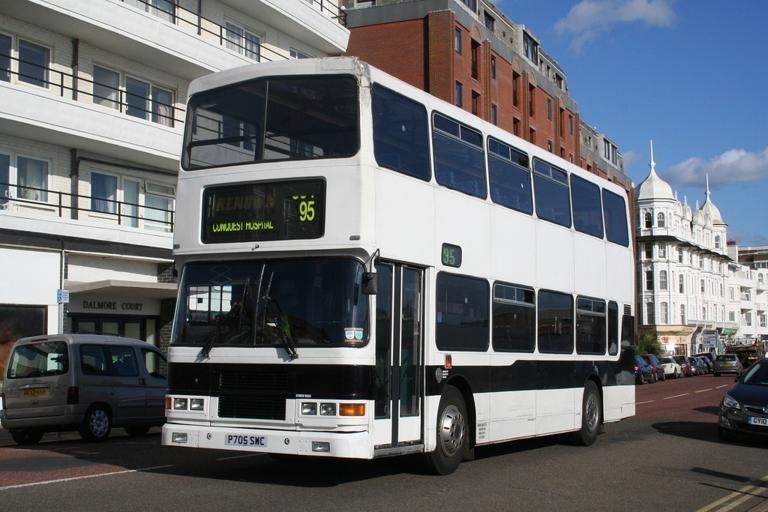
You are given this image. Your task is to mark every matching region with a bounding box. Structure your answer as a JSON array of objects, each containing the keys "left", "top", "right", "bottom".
[{"left": 161, "top": 55, "right": 636, "bottom": 474}]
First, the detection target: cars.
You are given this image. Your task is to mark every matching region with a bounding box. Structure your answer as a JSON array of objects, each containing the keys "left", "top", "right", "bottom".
[
  {"left": 635, "top": 352, "right": 743, "bottom": 384},
  {"left": 718, "top": 358, "right": 768, "bottom": 442}
]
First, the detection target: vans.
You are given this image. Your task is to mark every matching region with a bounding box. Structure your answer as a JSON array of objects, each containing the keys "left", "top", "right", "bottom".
[{"left": 0, "top": 333, "right": 167, "bottom": 442}]
